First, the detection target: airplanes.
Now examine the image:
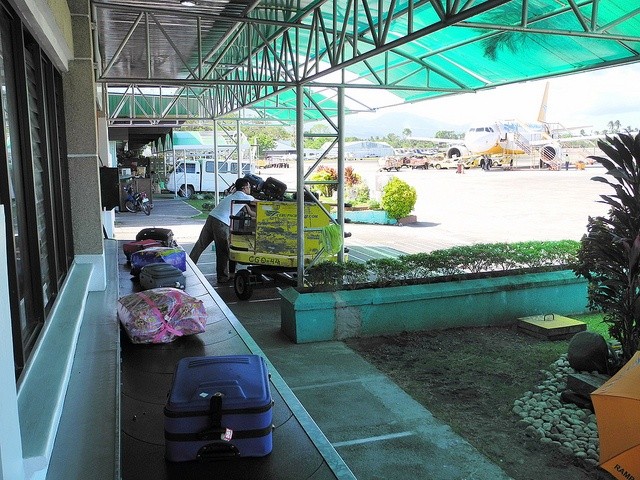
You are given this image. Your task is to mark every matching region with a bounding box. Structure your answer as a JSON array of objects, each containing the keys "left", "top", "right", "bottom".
[{"left": 408, "top": 82, "right": 640, "bottom": 171}]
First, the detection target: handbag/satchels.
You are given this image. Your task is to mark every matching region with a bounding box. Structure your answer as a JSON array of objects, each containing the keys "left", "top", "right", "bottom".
[
  {"left": 130, "top": 247, "right": 186, "bottom": 276},
  {"left": 117, "top": 287, "right": 206, "bottom": 344}
]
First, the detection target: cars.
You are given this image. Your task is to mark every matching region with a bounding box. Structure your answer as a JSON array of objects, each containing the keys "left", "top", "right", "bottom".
[{"left": 434, "top": 157, "right": 470, "bottom": 169}]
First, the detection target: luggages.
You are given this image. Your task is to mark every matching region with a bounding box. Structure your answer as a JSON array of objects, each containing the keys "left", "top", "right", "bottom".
[
  {"left": 140, "top": 262, "right": 186, "bottom": 288},
  {"left": 163, "top": 354, "right": 273, "bottom": 463},
  {"left": 137, "top": 228, "right": 174, "bottom": 247},
  {"left": 123, "top": 239, "right": 160, "bottom": 260},
  {"left": 262, "top": 177, "right": 287, "bottom": 200},
  {"left": 244, "top": 173, "right": 264, "bottom": 193}
]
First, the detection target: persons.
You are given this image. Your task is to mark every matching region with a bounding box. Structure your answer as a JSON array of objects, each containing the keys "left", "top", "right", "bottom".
[
  {"left": 412, "top": 154, "right": 429, "bottom": 170},
  {"left": 564, "top": 153, "right": 570, "bottom": 170},
  {"left": 480, "top": 154, "right": 492, "bottom": 170},
  {"left": 188, "top": 178, "right": 261, "bottom": 284}
]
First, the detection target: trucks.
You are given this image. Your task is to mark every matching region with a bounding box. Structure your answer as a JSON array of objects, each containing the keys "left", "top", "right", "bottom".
[
  {"left": 378, "top": 156, "right": 402, "bottom": 172},
  {"left": 164, "top": 158, "right": 255, "bottom": 198}
]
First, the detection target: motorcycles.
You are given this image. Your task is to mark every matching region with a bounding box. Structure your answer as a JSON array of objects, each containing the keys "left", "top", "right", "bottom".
[{"left": 124, "top": 182, "right": 153, "bottom": 215}]
